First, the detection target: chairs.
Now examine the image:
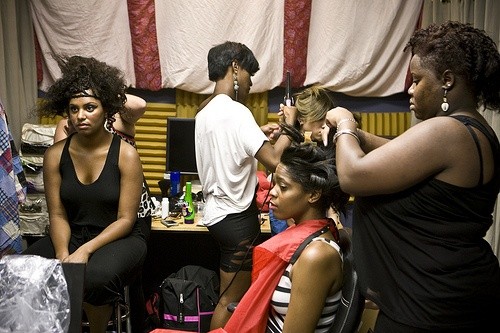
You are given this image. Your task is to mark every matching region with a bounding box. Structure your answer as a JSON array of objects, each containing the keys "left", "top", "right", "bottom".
[{"left": 332, "top": 258, "right": 367, "bottom": 333}]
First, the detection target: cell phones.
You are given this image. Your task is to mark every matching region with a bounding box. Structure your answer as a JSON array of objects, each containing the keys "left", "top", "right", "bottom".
[{"left": 161, "top": 219, "right": 178, "bottom": 227}]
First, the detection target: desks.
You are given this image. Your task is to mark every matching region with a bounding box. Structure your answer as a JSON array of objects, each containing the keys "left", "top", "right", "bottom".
[{"left": 151, "top": 207, "right": 343, "bottom": 233}]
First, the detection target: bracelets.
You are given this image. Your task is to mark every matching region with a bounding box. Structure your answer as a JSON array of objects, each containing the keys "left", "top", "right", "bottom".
[{"left": 332, "top": 118, "right": 361, "bottom": 144}]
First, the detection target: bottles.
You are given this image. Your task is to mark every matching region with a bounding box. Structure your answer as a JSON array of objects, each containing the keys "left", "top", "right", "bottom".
[
  {"left": 164, "top": 170, "right": 181, "bottom": 197},
  {"left": 182, "top": 180, "right": 195, "bottom": 223},
  {"left": 161, "top": 197, "right": 170, "bottom": 219}
]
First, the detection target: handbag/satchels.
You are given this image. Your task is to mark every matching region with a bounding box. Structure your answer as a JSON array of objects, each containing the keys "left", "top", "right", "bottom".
[{"left": 145, "top": 265, "right": 220, "bottom": 333}]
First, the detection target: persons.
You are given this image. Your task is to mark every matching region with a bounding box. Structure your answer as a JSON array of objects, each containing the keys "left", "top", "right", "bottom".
[
  {"left": 326, "top": 20, "right": 500, "bottom": 333},
  {"left": 24, "top": 55, "right": 153, "bottom": 333},
  {"left": 194, "top": 41, "right": 298, "bottom": 329},
  {"left": 149, "top": 128, "right": 344, "bottom": 333},
  {"left": 268, "top": 82, "right": 346, "bottom": 237}
]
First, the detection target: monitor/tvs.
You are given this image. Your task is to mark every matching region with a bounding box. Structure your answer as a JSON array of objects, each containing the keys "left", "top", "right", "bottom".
[{"left": 165, "top": 117, "right": 198, "bottom": 176}]
